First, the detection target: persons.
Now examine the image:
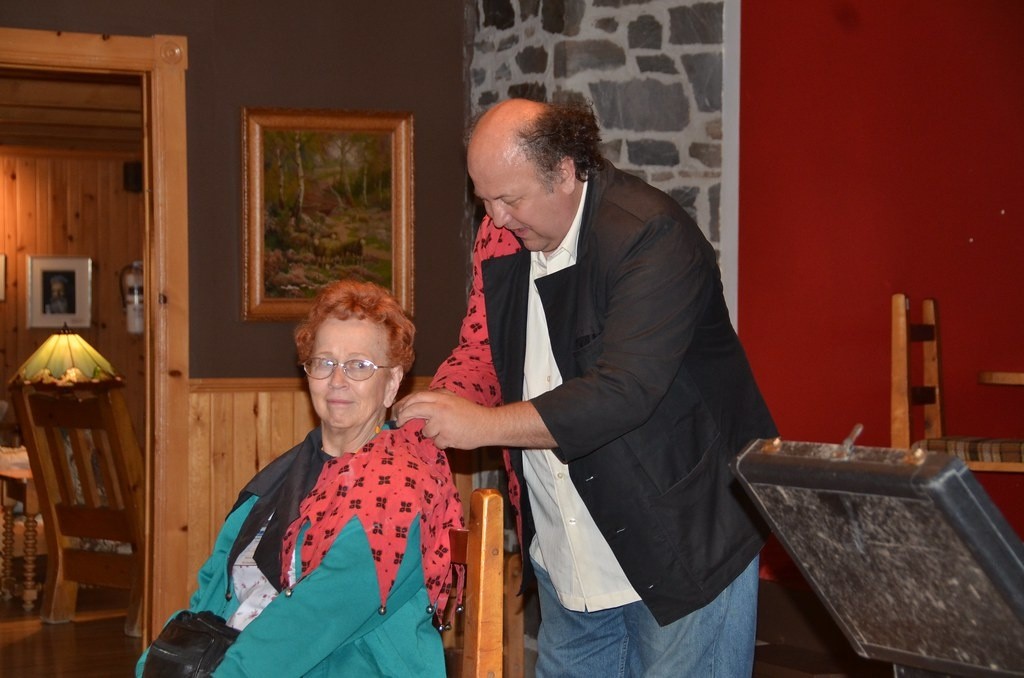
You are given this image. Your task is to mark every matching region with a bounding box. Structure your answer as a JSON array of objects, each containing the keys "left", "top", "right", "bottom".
[
  {"left": 392, "top": 96, "right": 779, "bottom": 678},
  {"left": 134, "top": 281, "right": 469, "bottom": 678},
  {"left": 44, "top": 275, "right": 72, "bottom": 313}
]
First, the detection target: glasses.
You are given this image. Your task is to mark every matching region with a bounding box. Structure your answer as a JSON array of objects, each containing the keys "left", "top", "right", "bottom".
[{"left": 300, "top": 356, "right": 395, "bottom": 382}]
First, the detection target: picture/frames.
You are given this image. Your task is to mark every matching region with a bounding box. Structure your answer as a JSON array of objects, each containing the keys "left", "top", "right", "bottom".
[
  {"left": 238, "top": 104, "right": 416, "bottom": 322},
  {"left": 24, "top": 253, "right": 94, "bottom": 331}
]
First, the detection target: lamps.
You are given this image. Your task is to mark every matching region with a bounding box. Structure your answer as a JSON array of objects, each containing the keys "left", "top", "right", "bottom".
[{"left": 8, "top": 320, "right": 126, "bottom": 398}]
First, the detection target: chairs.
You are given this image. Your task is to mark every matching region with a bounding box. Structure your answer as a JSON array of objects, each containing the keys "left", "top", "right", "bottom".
[
  {"left": 731, "top": 433, "right": 1024, "bottom": 678},
  {"left": 890, "top": 292, "right": 1024, "bottom": 476},
  {"left": 8, "top": 375, "right": 145, "bottom": 638},
  {"left": 448, "top": 485, "right": 506, "bottom": 677}
]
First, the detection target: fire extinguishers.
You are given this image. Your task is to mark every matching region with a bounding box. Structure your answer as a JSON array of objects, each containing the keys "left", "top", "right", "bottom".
[{"left": 119, "top": 260, "right": 145, "bottom": 334}]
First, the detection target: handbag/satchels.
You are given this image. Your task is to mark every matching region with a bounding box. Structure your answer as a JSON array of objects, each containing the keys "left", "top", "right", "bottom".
[{"left": 139, "top": 612, "right": 242, "bottom": 678}]
